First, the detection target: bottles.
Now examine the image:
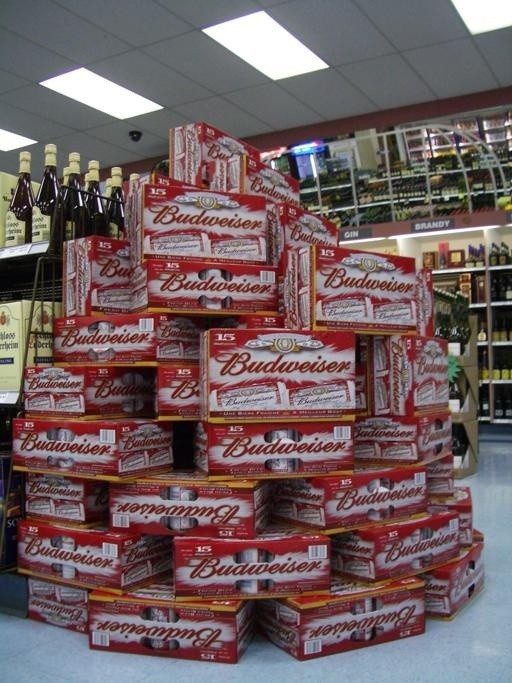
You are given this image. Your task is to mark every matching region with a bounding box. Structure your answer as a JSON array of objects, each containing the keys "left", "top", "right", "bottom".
[
  {"left": 296, "top": 144, "right": 512, "bottom": 227},
  {"left": 5, "top": 142, "right": 140, "bottom": 248},
  {"left": 439, "top": 243, "right": 448, "bottom": 270},
  {"left": 464, "top": 241, "right": 512, "bottom": 419},
  {"left": 450, "top": 374, "right": 466, "bottom": 410},
  {"left": 430, "top": 285, "right": 472, "bottom": 343},
  {"left": 452, "top": 423, "right": 464, "bottom": 472}
]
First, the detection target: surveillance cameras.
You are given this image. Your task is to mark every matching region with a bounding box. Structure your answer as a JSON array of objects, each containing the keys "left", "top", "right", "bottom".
[{"left": 129, "top": 131, "right": 142, "bottom": 142}]
